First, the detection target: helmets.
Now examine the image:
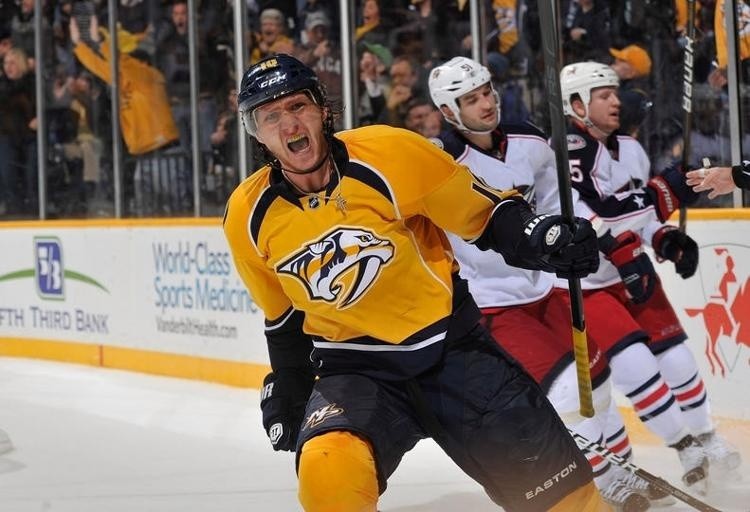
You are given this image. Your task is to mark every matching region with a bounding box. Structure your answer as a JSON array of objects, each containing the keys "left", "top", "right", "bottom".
[
  {"left": 427, "top": 56, "right": 496, "bottom": 118},
  {"left": 559, "top": 60, "right": 620, "bottom": 117},
  {"left": 237, "top": 53, "right": 328, "bottom": 134}
]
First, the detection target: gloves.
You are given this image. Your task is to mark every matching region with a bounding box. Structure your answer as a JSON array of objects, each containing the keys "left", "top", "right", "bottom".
[
  {"left": 652, "top": 224, "right": 700, "bottom": 280},
  {"left": 260, "top": 308, "right": 315, "bottom": 452},
  {"left": 604, "top": 230, "right": 656, "bottom": 304},
  {"left": 478, "top": 200, "right": 598, "bottom": 279},
  {"left": 646, "top": 165, "right": 702, "bottom": 224}
]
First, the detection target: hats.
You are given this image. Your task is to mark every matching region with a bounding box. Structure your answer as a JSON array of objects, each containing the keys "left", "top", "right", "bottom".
[
  {"left": 609, "top": 44, "right": 652, "bottom": 75},
  {"left": 360, "top": 41, "right": 393, "bottom": 69},
  {"left": 305, "top": 10, "right": 333, "bottom": 28},
  {"left": 104, "top": 30, "right": 137, "bottom": 53}
]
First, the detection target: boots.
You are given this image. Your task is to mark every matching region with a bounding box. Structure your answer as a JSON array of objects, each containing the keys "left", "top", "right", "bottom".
[
  {"left": 597, "top": 472, "right": 671, "bottom": 512},
  {"left": 663, "top": 427, "right": 710, "bottom": 487},
  {"left": 697, "top": 427, "right": 742, "bottom": 469}
]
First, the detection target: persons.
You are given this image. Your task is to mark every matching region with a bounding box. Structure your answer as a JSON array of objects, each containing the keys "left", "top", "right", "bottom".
[
  {"left": 547, "top": 61, "right": 741, "bottom": 485},
  {"left": 222, "top": 56, "right": 617, "bottom": 512},
  {"left": 1, "top": 0, "right": 750, "bottom": 218},
  {"left": 429, "top": 55, "right": 671, "bottom": 512}
]
